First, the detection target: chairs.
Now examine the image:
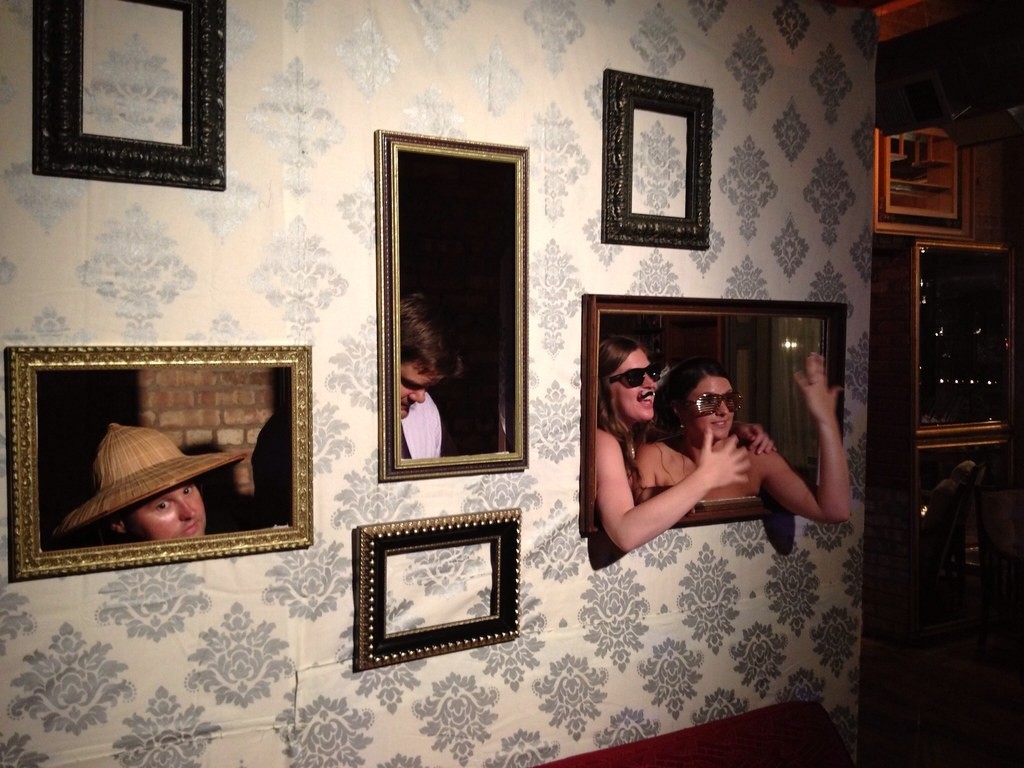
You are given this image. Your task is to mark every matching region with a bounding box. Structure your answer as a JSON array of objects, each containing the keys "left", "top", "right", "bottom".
[{"left": 974, "top": 484, "right": 1024, "bottom": 685}]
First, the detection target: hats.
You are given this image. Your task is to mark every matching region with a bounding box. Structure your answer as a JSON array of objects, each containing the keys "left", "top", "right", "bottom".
[{"left": 49, "top": 421, "right": 246, "bottom": 540}]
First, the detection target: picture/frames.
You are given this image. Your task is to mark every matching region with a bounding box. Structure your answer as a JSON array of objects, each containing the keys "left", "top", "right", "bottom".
[
  {"left": 32, "top": 0, "right": 227, "bottom": 192},
  {"left": 4, "top": 345, "right": 315, "bottom": 584},
  {"left": 578, "top": 295, "right": 848, "bottom": 537},
  {"left": 601, "top": 68, "right": 715, "bottom": 251},
  {"left": 373, "top": 130, "right": 529, "bottom": 483},
  {"left": 350, "top": 508, "right": 522, "bottom": 673}
]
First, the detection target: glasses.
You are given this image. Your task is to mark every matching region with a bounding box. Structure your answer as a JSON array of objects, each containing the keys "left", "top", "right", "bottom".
[
  {"left": 683, "top": 390, "right": 745, "bottom": 415},
  {"left": 609, "top": 361, "right": 662, "bottom": 389}
]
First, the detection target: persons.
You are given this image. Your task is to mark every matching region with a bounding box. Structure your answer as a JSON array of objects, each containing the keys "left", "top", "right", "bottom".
[
  {"left": 595, "top": 336, "right": 851, "bottom": 553},
  {"left": 54, "top": 424, "right": 246, "bottom": 540},
  {"left": 401, "top": 300, "right": 455, "bottom": 460}
]
